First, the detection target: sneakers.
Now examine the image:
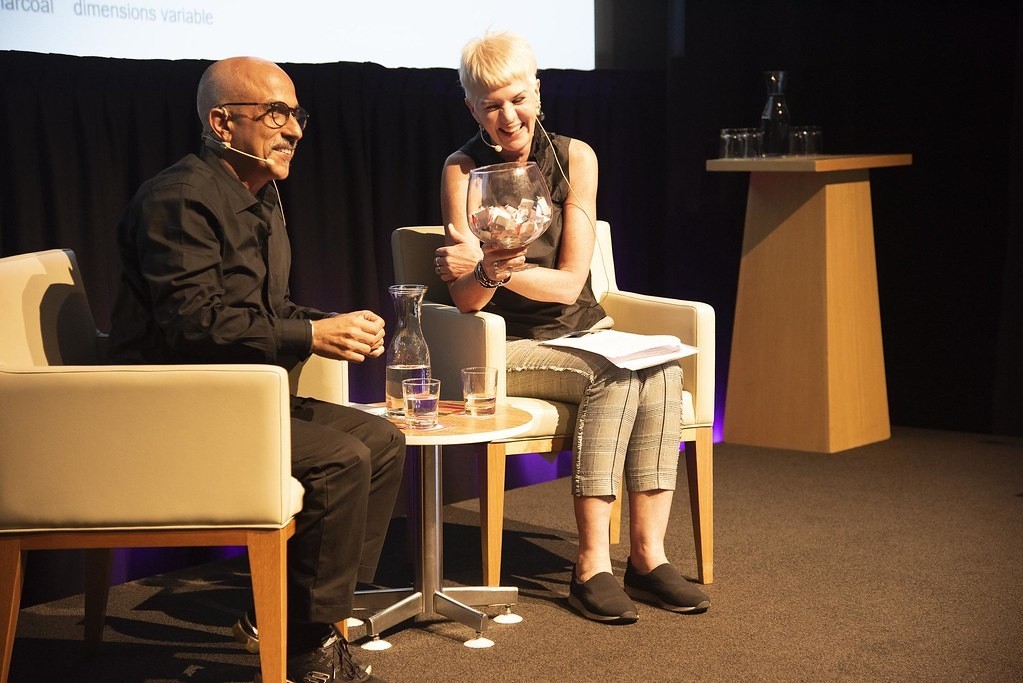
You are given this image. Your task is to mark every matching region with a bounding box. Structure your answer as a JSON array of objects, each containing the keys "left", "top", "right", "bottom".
[
  {"left": 566, "top": 561, "right": 640, "bottom": 622},
  {"left": 623, "top": 555, "right": 712, "bottom": 613},
  {"left": 284, "top": 621, "right": 387, "bottom": 683},
  {"left": 231, "top": 601, "right": 260, "bottom": 653}
]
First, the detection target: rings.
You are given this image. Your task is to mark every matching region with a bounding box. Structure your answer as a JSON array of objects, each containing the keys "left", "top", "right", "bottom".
[
  {"left": 438, "top": 266, "right": 441, "bottom": 276},
  {"left": 494, "top": 262, "right": 499, "bottom": 270},
  {"left": 434, "top": 256, "right": 440, "bottom": 267}
]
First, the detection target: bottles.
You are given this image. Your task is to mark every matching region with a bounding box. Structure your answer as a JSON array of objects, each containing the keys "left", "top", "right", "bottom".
[
  {"left": 386, "top": 283, "right": 429, "bottom": 419},
  {"left": 760, "top": 70, "right": 790, "bottom": 157}
]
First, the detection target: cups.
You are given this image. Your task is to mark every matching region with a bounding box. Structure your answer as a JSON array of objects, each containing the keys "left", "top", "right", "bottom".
[
  {"left": 789, "top": 125, "right": 809, "bottom": 156},
  {"left": 809, "top": 125, "right": 824, "bottom": 157},
  {"left": 460, "top": 366, "right": 497, "bottom": 418},
  {"left": 402, "top": 378, "right": 441, "bottom": 429},
  {"left": 717, "top": 127, "right": 764, "bottom": 160}
]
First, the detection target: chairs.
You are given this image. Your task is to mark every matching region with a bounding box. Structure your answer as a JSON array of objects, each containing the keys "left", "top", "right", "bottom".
[
  {"left": 0, "top": 246, "right": 350, "bottom": 683},
  {"left": 387, "top": 219, "right": 717, "bottom": 604}
]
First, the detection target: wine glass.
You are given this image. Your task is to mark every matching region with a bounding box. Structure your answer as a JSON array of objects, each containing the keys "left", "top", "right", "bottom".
[{"left": 467, "top": 160, "right": 554, "bottom": 273}]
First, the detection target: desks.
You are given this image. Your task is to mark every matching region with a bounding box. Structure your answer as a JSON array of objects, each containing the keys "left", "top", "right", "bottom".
[
  {"left": 345, "top": 399, "right": 535, "bottom": 653},
  {"left": 704, "top": 153, "right": 913, "bottom": 456}
]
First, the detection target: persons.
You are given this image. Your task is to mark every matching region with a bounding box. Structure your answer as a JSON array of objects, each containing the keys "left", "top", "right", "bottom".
[
  {"left": 109, "top": 58, "right": 404, "bottom": 683},
  {"left": 435, "top": 31, "right": 713, "bottom": 622}
]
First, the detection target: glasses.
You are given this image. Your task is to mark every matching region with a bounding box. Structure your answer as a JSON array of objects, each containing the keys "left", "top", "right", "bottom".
[{"left": 218, "top": 100, "right": 309, "bottom": 130}]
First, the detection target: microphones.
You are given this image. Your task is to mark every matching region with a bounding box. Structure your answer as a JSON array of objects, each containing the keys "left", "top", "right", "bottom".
[
  {"left": 479, "top": 124, "right": 503, "bottom": 153},
  {"left": 219, "top": 141, "right": 275, "bottom": 165}
]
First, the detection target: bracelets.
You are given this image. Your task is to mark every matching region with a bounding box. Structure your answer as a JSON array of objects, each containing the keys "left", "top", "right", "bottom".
[{"left": 474, "top": 262, "right": 511, "bottom": 288}]
